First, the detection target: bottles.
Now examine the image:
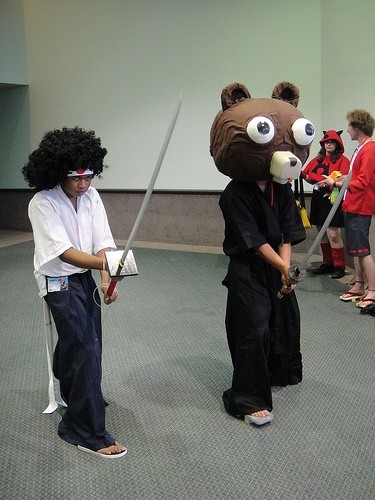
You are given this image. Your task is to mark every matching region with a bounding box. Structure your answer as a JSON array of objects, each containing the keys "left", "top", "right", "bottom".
[{"left": 331, "top": 187, "right": 340, "bottom": 204}]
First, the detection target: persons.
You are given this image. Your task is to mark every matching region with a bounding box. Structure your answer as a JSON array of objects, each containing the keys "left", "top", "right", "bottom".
[
  {"left": 339, "top": 110, "right": 375, "bottom": 308},
  {"left": 22, "top": 126, "right": 126, "bottom": 459},
  {"left": 302, "top": 129, "right": 350, "bottom": 280},
  {"left": 211, "top": 81, "right": 316, "bottom": 429}
]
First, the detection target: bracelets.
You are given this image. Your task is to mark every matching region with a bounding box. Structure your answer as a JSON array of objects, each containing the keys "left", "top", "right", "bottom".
[{"left": 333, "top": 181, "right": 336, "bottom": 188}]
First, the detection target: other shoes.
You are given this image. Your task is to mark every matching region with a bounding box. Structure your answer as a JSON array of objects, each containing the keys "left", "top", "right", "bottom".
[
  {"left": 311, "top": 264, "right": 333, "bottom": 274},
  {"left": 332, "top": 270, "right": 345, "bottom": 279}
]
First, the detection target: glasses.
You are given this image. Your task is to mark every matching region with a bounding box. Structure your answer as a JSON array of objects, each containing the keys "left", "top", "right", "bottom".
[{"left": 325, "top": 140, "right": 335, "bottom": 145}]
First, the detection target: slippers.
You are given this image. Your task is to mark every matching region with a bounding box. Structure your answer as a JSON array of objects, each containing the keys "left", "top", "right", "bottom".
[
  {"left": 78, "top": 439, "right": 127, "bottom": 458},
  {"left": 244, "top": 409, "right": 273, "bottom": 426},
  {"left": 357, "top": 297, "right": 375, "bottom": 308},
  {"left": 340, "top": 289, "right": 365, "bottom": 302}
]
